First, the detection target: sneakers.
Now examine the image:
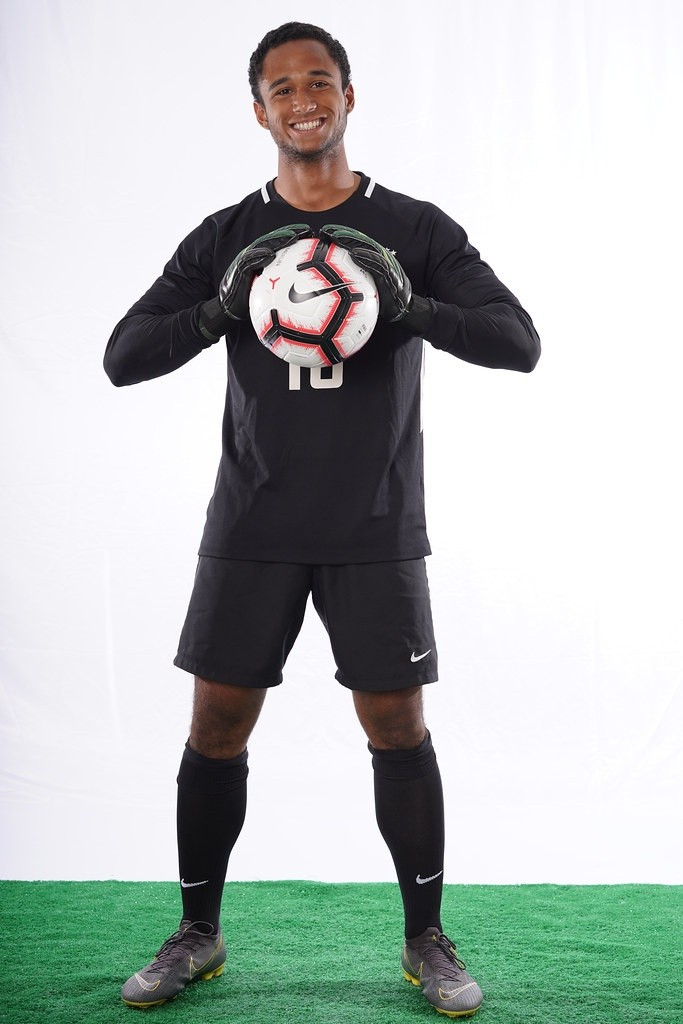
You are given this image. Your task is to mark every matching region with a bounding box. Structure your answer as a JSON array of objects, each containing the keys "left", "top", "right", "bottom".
[
  {"left": 401, "top": 927, "right": 483, "bottom": 1019},
  {"left": 120, "top": 917, "right": 228, "bottom": 1008}
]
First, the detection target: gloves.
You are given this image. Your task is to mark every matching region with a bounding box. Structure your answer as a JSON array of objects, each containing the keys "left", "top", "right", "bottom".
[
  {"left": 317, "top": 219, "right": 433, "bottom": 335},
  {"left": 192, "top": 223, "right": 315, "bottom": 339}
]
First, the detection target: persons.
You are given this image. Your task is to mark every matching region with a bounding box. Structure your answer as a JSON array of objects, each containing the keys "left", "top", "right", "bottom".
[{"left": 102, "top": 21, "right": 543, "bottom": 1016}]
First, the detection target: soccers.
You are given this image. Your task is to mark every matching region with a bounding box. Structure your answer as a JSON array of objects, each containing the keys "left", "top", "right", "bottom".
[{"left": 250, "top": 237, "right": 380, "bottom": 366}]
